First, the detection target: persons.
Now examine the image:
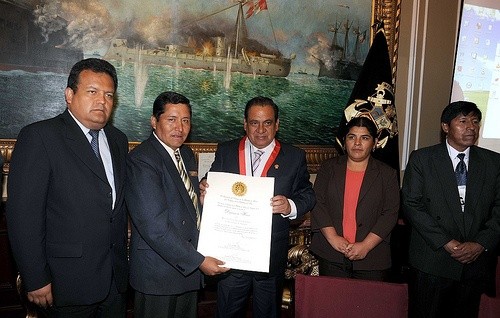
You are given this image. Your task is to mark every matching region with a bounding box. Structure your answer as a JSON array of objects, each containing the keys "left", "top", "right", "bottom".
[
  {"left": 401, "top": 100, "right": 500, "bottom": 318},
  {"left": 199, "top": 96, "right": 316, "bottom": 318},
  {"left": 6, "top": 58, "right": 128, "bottom": 318},
  {"left": 308, "top": 117, "right": 400, "bottom": 281},
  {"left": 124, "top": 91, "right": 224, "bottom": 318}
]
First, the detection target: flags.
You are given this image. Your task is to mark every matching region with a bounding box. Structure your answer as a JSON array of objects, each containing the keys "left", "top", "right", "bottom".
[
  {"left": 335, "top": 20, "right": 400, "bottom": 186},
  {"left": 245, "top": 0, "right": 268, "bottom": 20}
]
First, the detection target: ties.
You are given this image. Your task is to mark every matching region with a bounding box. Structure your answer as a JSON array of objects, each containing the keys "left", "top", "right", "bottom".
[
  {"left": 455, "top": 153, "right": 470, "bottom": 209},
  {"left": 249, "top": 148, "right": 264, "bottom": 175},
  {"left": 173, "top": 149, "right": 203, "bottom": 230},
  {"left": 87, "top": 129, "right": 101, "bottom": 158}
]
